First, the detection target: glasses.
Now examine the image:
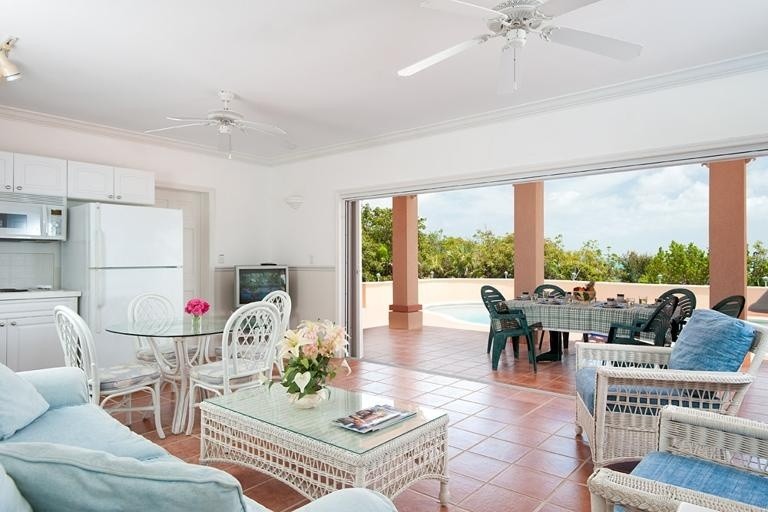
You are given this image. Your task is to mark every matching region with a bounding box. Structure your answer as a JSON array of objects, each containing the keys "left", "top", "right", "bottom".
[
  {"left": 0, "top": 40, "right": 21, "bottom": 84},
  {"left": 506, "top": 30, "right": 527, "bottom": 90}
]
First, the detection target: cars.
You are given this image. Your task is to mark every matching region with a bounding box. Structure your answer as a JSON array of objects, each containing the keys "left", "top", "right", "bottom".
[
  {"left": 345, "top": 389, "right": 360, "bottom": 417},
  {"left": 638, "top": 297, "right": 647, "bottom": 307},
  {"left": 520, "top": 292, "right": 562, "bottom": 304},
  {"left": 603, "top": 293, "right": 635, "bottom": 308}
]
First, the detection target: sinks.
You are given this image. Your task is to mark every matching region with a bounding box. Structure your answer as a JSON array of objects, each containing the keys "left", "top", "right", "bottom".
[{"left": 1, "top": 368, "right": 400, "bottom": 512}]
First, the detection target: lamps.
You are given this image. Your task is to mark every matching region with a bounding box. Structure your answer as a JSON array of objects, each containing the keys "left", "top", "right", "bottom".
[
  {"left": 262, "top": 288, "right": 291, "bottom": 342},
  {"left": 480, "top": 283, "right": 745, "bottom": 369},
  {"left": 588, "top": 400, "right": 768, "bottom": 512},
  {"left": 185, "top": 301, "right": 285, "bottom": 435},
  {"left": 52, "top": 304, "right": 165, "bottom": 439},
  {"left": 569, "top": 303, "right": 768, "bottom": 471},
  {"left": 127, "top": 292, "right": 202, "bottom": 363}
]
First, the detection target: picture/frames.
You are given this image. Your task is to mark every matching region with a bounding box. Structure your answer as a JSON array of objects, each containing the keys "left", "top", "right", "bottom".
[
  {"left": 197, "top": 377, "right": 460, "bottom": 508},
  {"left": 102, "top": 317, "right": 271, "bottom": 436}
]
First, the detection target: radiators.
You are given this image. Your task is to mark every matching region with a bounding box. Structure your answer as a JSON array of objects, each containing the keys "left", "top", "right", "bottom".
[{"left": 261, "top": 263, "right": 276, "bottom": 265}]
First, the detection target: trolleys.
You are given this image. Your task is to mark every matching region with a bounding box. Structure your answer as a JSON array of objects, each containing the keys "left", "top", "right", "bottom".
[
  {"left": 396, "top": 0, "right": 643, "bottom": 76},
  {"left": 143, "top": 88, "right": 292, "bottom": 159}
]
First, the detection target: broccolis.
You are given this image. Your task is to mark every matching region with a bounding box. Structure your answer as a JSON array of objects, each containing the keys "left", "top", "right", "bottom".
[{"left": 62, "top": 202, "right": 187, "bottom": 385}]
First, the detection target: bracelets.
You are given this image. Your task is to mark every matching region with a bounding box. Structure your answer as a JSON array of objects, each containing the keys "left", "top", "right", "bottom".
[{"left": 573, "top": 291, "right": 596, "bottom": 304}]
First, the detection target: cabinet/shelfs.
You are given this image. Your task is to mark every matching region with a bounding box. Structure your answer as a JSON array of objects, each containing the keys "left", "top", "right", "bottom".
[{"left": 565, "top": 291, "right": 572, "bottom": 305}]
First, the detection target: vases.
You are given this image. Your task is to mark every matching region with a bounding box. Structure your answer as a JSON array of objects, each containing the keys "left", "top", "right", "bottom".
[
  {"left": 183, "top": 298, "right": 211, "bottom": 316},
  {"left": 275, "top": 314, "right": 354, "bottom": 406}
]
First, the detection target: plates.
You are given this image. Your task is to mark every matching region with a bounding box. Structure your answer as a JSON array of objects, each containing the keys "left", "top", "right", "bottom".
[
  {"left": 69, "top": 163, "right": 156, "bottom": 204},
  {"left": 1, "top": 151, "right": 68, "bottom": 202},
  {"left": 0, "top": 292, "right": 83, "bottom": 370}
]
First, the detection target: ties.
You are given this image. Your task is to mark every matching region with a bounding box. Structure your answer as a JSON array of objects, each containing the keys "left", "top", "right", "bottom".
[{"left": 234, "top": 265, "right": 289, "bottom": 307}]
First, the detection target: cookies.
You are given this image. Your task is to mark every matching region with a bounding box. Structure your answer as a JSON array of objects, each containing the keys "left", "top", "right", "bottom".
[{"left": 1, "top": 202, "right": 67, "bottom": 241}]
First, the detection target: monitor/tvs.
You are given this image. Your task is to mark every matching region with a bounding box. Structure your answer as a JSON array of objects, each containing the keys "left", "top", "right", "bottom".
[{"left": 328, "top": 404, "right": 417, "bottom": 434}]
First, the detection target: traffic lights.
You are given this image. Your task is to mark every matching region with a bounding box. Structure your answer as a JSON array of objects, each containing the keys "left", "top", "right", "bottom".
[
  {"left": 2, "top": 436, "right": 248, "bottom": 512},
  {"left": 1, "top": 365, "right": 53, "bottom": 433}
]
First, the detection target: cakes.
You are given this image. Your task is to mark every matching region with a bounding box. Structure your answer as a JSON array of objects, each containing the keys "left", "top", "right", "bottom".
[
  {"left": 548, "top": 296, "right": 554, "bottom": 303},
  {"left": 607, "top": 298, "right": 614, "bottom": 306},
  {"left": 617, "top": 294, "right": 624, "bottom": 303},
  {"left": 522, "top": 291, "right": 529, "bottom": 300}
]
